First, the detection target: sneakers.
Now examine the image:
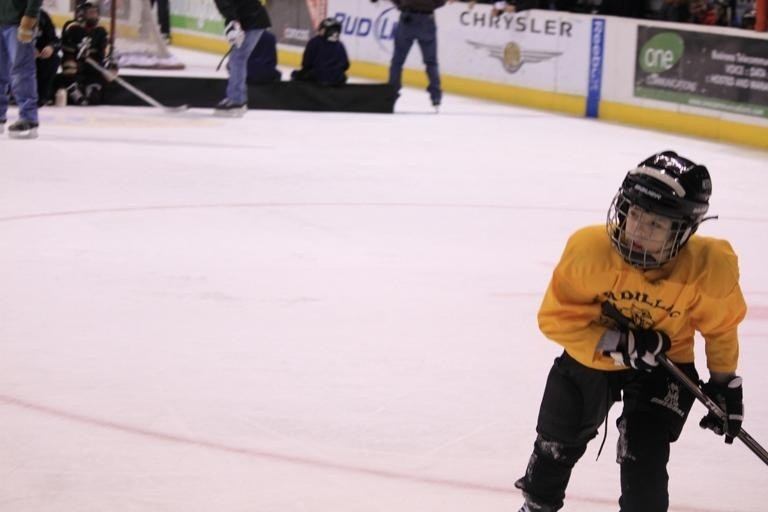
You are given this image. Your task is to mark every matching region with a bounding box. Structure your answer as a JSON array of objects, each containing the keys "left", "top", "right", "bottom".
[
  {"left": 8, "top": 121, "right": 39, "bottom": 131},
  {"left": 216, "top": 97, "right": 248, "bottom": 109},
  {"left": 513, "top": 477, "right": 556, "bottom": 512}
]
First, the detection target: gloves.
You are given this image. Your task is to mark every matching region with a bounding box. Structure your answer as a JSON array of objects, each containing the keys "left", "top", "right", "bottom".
[
  {"left": 699, "top": 377, "right": 742, "bottom": 444},
  {"left": 616, "top": 328, "right": 670, "bottom": 373},
  {"left": 223, "top": 19, "right": 245, "bottom": 48}
]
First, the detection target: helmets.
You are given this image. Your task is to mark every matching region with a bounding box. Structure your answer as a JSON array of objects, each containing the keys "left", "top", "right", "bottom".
[
  {"left": 74, "top": 0, "right": 100, "bottom": 27},
  {"left": 318, "top": 17, "right": 341, "bottom": 40},
  {"left": 605, "top": 150, "right": 712, "bottom": 270}
]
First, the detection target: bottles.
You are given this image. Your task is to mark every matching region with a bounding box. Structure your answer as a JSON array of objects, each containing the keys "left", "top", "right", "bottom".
[{"left": 56, "top": 89, "right": 67, "bottom": 106}]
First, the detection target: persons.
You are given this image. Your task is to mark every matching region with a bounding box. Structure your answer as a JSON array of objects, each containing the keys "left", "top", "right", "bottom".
[
  {"left": 290, "top": 18, "right": 350, "bottom": 80},
  {"left": 214, "top": 1, "right": 282, "bottom": 115},
  {"left": 504, "top": 2, "right": 768, "bottom": 32},
  {"left": 36, "top": 1, "right": 120, "bottom": 105},
  {"left": 150, "top": 1, "right": 172, "bottom": 43},
  {"left": 1, "top": 1, "right": 44, "bottom": 136},
  {"left": 513, "top": 149, "right": 743, "bottom": 512},
  {"left": 389, "top": 1, "right": 447, "bottom": 114}
]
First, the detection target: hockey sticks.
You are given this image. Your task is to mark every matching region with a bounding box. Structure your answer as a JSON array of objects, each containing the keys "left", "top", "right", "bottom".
[{"left": 86, "top": 58, "right": 188, "bottom": 112}]
